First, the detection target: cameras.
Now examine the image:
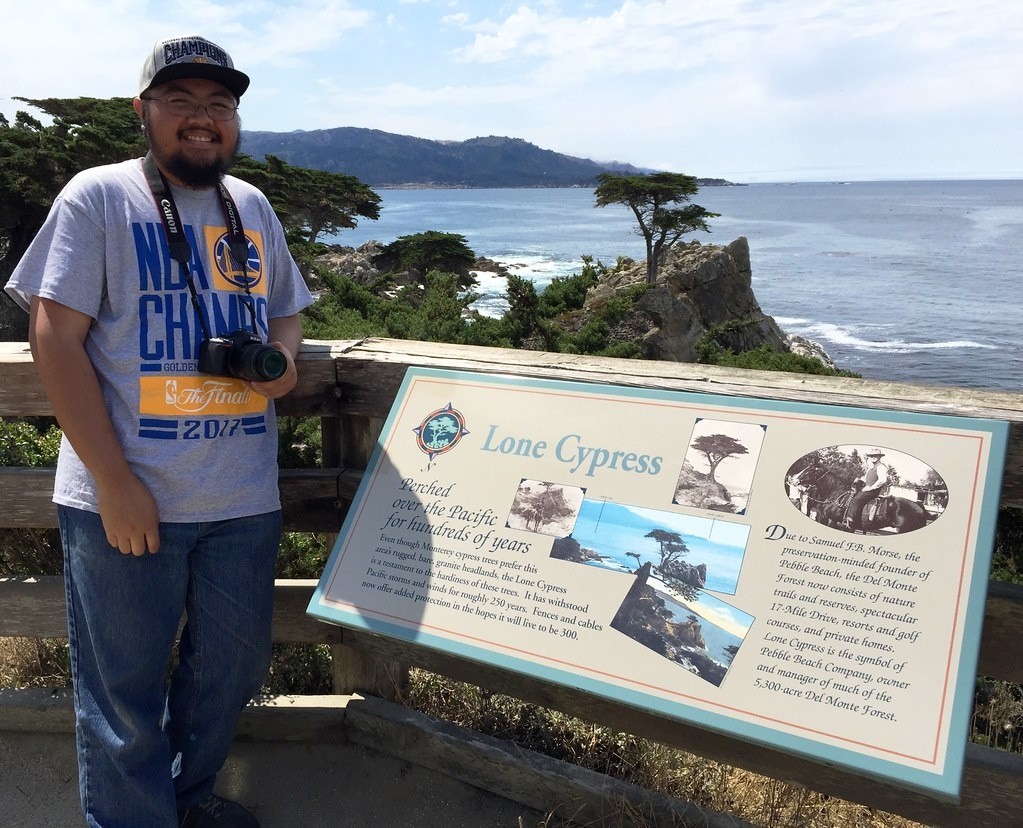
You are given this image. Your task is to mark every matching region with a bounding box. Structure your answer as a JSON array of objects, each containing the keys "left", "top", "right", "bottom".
[{"left": 196, "top": 329, "right": 287, "bottom": 382}]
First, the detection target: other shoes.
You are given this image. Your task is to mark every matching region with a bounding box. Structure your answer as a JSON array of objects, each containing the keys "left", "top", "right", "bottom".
[
  {"left": 837, "top": 521, "right": 855, "bottom": 532},
  {"left": 178, "top": 794, "right": 260, "bottom": 828}
]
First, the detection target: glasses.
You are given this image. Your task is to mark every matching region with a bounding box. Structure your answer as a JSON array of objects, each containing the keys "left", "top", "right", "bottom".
[{"left": 140, "top": 94, "right": 239, "bottom": 122}]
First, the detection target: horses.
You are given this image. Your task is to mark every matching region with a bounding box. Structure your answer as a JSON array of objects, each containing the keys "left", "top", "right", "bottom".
[{"left": 793, "top": 457, "right": 926, "bottom": 535}]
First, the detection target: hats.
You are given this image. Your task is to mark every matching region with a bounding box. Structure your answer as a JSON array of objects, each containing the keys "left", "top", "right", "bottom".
[
  {"left": 867, "top": 449, "right": 885, "bottom": 456},
  {"left": 136, "top": 34, "right": 250, "bottom": 100}
]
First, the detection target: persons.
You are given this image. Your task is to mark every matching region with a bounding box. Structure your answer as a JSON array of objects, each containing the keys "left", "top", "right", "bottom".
[
  {"left": 4, "top": 37, "right": 314, "bottom": 828},
  {"left": 837, "top": 448, "right": 888, "bottom": 534},
  {"left": 786, "top": 475, "right": 812, "bottom": 514}
]
